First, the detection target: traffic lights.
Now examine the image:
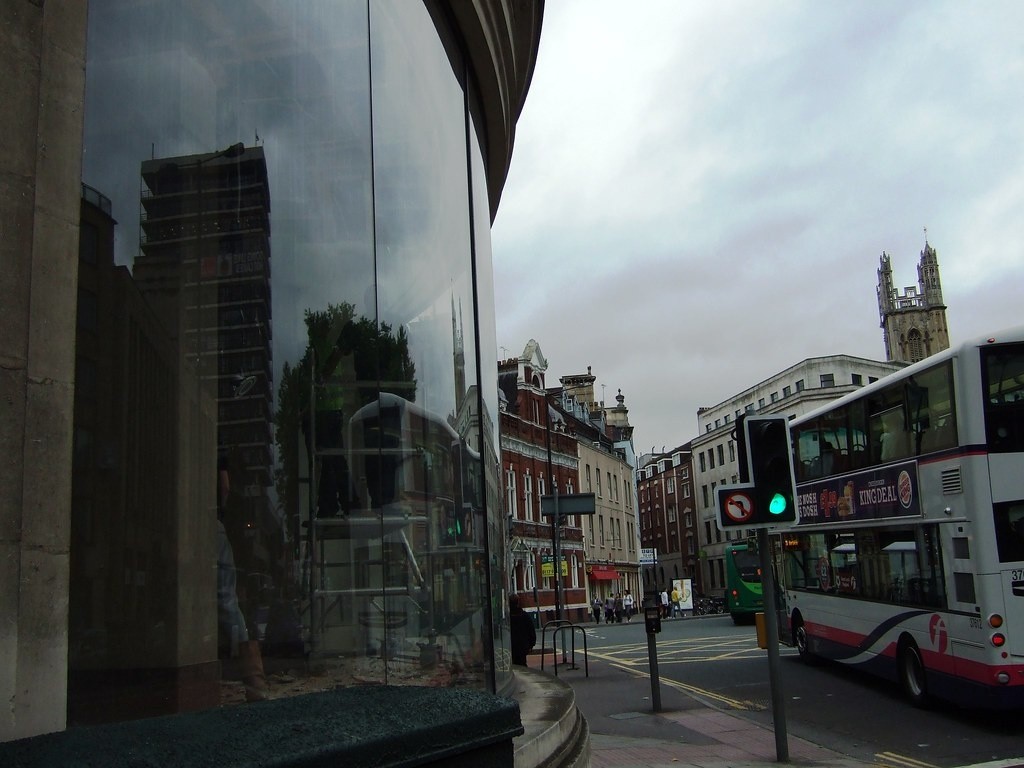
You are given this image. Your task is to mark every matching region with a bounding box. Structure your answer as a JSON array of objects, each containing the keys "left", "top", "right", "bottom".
[{"left": 746, "top": 410, "right": 801, "bottom": 528}]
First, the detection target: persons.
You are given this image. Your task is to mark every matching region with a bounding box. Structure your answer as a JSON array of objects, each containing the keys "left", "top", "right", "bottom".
[
  {"left": 606, "top": 590, "right": 634, "bottom": 624},
  {"left": 509, "top": 593, "right": 536, "bottom": 668},
  {"left": 671, "top": 586, "right": 687, "bottom": 619},
  {"left": 591, "top": 593, "right": 603, "bottom": 625},
  {"left": 660, "top": 587, "right": 672, "bottom": 620},
  {"left": 843, "top": 479, "right": 855, "bottom": 498},
  {"left": 879, "top": 415, "right": 901, "bottom": 463},
  {"left": 218, "top": 433, "right": 270, "bottom": 701}
]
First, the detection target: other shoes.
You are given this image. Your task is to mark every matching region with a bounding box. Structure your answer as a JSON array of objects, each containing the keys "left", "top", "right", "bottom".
[
  {"left": 626, "top": 620, "right": 628, "bottom": 623},
  {"left": 672, "top": 616, "right": 675, "bottom": 619},
  {"left": 667, "top": 617, "right": 672, "bottom": 619},
  {"left": 628, "top": 619, "right": 631, "bottom": 622},
  {"left": 682, "top": 614, "right": 686, "bottom": 617}
]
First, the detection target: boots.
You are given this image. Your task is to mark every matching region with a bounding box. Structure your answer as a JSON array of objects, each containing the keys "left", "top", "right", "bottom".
[{"left": 238, "top": 639, "right": 267, "bottom": 702}]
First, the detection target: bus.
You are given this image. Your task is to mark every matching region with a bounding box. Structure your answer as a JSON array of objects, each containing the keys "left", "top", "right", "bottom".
[
  {"left": 726, "top": 535, "right": 794, "bottom": 624},
  {"left": 731, "top": 324, "right": 1024, "bottom": 711}
]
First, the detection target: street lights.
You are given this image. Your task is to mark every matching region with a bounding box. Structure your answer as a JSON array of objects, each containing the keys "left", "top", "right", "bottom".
[{"left": 547, "top": 375, "right": 596, "bottom": 660}]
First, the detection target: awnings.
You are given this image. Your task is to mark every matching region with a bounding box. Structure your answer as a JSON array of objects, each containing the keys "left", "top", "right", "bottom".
[{"left": 589, "top": 565, "right": 621, "bottom": 580}]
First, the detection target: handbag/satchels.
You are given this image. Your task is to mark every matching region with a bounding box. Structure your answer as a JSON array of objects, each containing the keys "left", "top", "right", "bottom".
[
  {"left": 597, "top": 599, "right": 604, "bottom": 607},
  {"left": 631, "top": 605, "right": 633, "bottom": 609}
]
